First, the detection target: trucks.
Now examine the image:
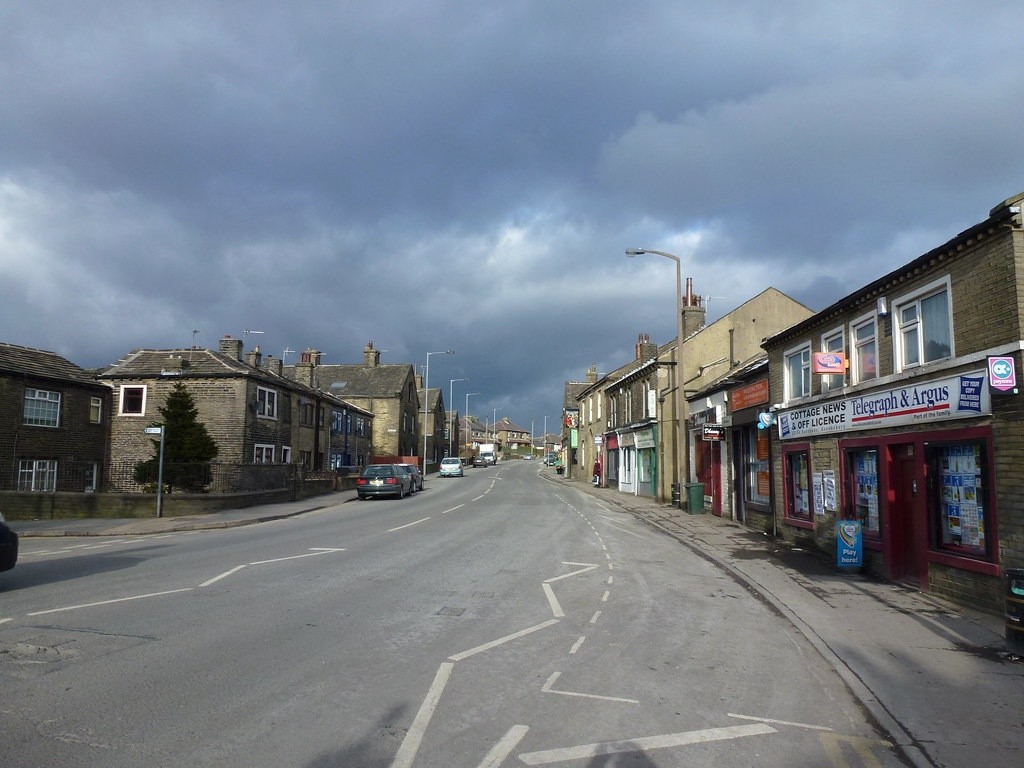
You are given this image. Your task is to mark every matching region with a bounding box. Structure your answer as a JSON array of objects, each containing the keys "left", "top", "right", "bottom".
[{"left": 480, "top": 444, "right": 497, "bottom": 465}]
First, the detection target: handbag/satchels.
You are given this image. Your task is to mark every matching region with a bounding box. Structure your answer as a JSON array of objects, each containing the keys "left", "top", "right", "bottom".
[{"left": 591, "top": 476, "right": 599, "bottom": 485}]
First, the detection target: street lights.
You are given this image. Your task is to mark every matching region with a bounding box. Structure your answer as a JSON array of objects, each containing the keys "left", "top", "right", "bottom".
[
  {"left": 494, "top": 408, "right": 502, "bottom": 444},
  {"left": 450, "top": 378, "right": 470, "bottom": 457},
  {"left": 625, "top": 247, "right": 687, "bottom": 508},
  {"left": 423, "top": 350, "right": 455, "bottom": 475},
  {"left": 466, "top": 392, "right": 482, "bottom": 452},
  {"left": 544, "top": 416, "right": 551, "bottom": 448}
]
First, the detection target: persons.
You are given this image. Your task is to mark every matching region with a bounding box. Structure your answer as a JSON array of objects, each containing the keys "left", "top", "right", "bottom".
[{"left": 593, "top": 459, "right": 600, "bottom": 487}]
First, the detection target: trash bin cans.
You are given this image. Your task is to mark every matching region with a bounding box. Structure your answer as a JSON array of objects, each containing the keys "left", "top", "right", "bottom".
[
  {"left": 557, "top": 469, "right": 562, "bottom": 474},
  {"left": 1003, "top": 568, "right": 1024, "bottom": 656},
  {"left": 671, "top": 483, "right": 680, "bottom": 507},
  {"left": 684, "top": 483, "right": 705, "bottom": 514}
]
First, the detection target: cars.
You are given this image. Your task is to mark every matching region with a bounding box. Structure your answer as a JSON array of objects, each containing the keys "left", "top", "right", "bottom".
[
  {"left": 440, "top": 457, "right": 463, "bottom": 477},
  {"left": 387, "top": 464, "right": 424, "bottom": 493},
  {"left": 355, "top": 464, "right": 411, "bottom": 501},
  {"left": 473, "top": 456, "right": 487, "bottom": 468},
  {"left": 524, "top": 453, "right": 535, "bottom": 460},
  {"left": 544, "top": 454, "right": 554, "bottom": 464},
  {"left": 547, "top": 455, "right": 555, "bottom": 465}
]
